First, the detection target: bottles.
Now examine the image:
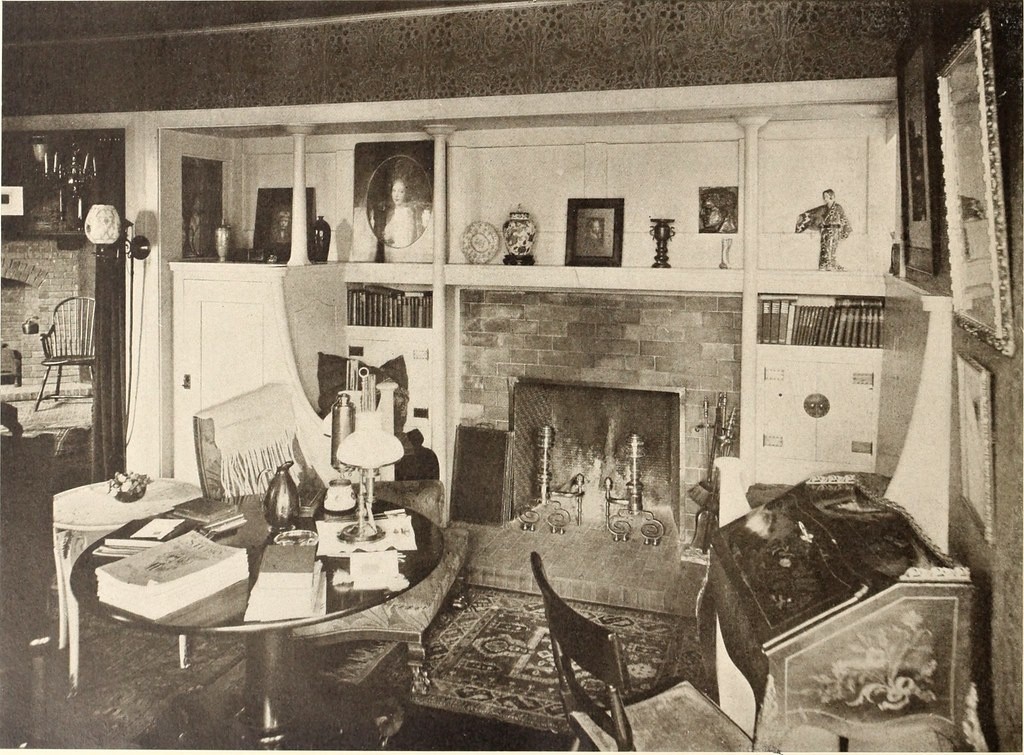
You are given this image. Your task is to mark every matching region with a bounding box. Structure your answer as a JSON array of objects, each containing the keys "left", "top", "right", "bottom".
[
  {"left": 502, "top": 204, "right": 536, "bottom": 256},
  {"left": 310, "top": 216, "right": 331, "bottom": 261},
  {"left": 216, "top": 219, "right": 231, "bottom": 261},
  {"left": 21, "top": 136, "right": 56, "bottom": 230}
]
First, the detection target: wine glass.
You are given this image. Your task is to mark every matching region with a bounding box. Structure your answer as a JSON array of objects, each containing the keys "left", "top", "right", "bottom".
[
  {"left": 66, "top": 154, "right": 97, "bottom": 231},
  {"left": 40, "top": 152, "right": 72, "bottom": 231}
]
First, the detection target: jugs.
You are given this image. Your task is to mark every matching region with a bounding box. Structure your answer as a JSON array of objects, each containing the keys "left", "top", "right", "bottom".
[{"left": 257, "top": 461, "right": 299, "bottom": 534}]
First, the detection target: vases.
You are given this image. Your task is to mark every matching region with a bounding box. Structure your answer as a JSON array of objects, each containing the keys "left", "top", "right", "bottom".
[
  {"left": 503, "top": 212, "right": 536, "bottom": 255},
  {"left": 214, "top": 218, "right": 230, "bottom": 263},
  {"left": 311, "top": 215, "right": 331, "bottom": 262}
]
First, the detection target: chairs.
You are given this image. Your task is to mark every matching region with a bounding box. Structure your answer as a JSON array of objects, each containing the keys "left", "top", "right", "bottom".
[
  {"left": 529, "top": 551, "right": 753, "bottom": 752},
  {"left": 194, "top": 381, "right": 469, "bottom": 697},
  {"left": 34, "top": 296, "right": 95, "bottom": 410}
]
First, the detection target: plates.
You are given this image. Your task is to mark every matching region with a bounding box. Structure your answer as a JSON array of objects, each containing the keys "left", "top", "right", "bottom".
[{"left": 461, "top": 222, "right": 500, "bottom": 265}]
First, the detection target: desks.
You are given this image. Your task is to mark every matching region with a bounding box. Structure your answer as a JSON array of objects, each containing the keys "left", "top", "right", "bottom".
[
  {"left": 69, "top": 490, "right": 443, "bottom": 750},
  {"left": 53, "top": 476, "right": 203, "bottom": 699}
]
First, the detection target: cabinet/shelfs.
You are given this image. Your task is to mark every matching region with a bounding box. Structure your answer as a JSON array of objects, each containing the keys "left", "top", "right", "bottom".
[{"left": 157, "top": 101, "right": 896, "bottom": 522}]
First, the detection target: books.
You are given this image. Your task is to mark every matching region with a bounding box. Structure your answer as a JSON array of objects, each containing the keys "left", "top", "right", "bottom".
[
  {"left": 91, "top": 517, "right": 195, "bottom": 556},
  {"left": 95, "top": 528, "right": 250, "bottom": 621},
  {"left": 242, "top": 544, "right": 327, "bottom": 623},
  {"left": 169, "top": 495, "right": 247, "bottom": 532},
  {"left": 758, "top": 295, "right": 882, "bottom": 347},
  {"left": 346, "top": 284, "right": 433, "bottom": 327}
]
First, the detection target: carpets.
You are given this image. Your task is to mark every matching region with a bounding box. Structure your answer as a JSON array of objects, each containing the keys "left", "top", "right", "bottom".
[{"left": 318, "top": 586, "right": 708, "bottom": 737}]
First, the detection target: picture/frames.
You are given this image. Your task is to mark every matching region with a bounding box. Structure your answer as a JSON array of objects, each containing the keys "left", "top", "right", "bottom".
[
  {"left": 952, "top": 347, "right": 993, "bottom": 545},
  {"left": 894, "top": 9, "right": 1016, "bottom": 360},
  {"left": 254, "top": 187, "right": 316, "bottom": 262},
  {"left": 347, "top": 140, "right": 448, "bottom": 263},
  {"left": 565, "top": 198, "right": 624, "bottom": 267}
]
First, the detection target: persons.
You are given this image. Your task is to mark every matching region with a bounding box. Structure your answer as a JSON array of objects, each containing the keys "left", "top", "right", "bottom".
[
  {"left": 273, "top": 211, "right": 291, "bottom": 242},
  {"left": 383, "top": 177, "right": 418, "bottom": 244},
  {"left": 794, "top": 188, "right": 852, "bottom": 272},
  {"left": 583, "top": 219, "right": 601, "bottom": 246},
  {"left": 699, "top": 188, "right": 737, "bottom": 233}
]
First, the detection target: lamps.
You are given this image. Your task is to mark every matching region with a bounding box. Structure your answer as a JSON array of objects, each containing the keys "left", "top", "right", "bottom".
[
  {"left": 85, "top": 203, "right": 151, "bottom": 261},
  {"left": 336, "top": 410, "right": 405, "bottom": 547},
  {"left": 29, "top": 134, "right": 97, "bottom": 231}
]
